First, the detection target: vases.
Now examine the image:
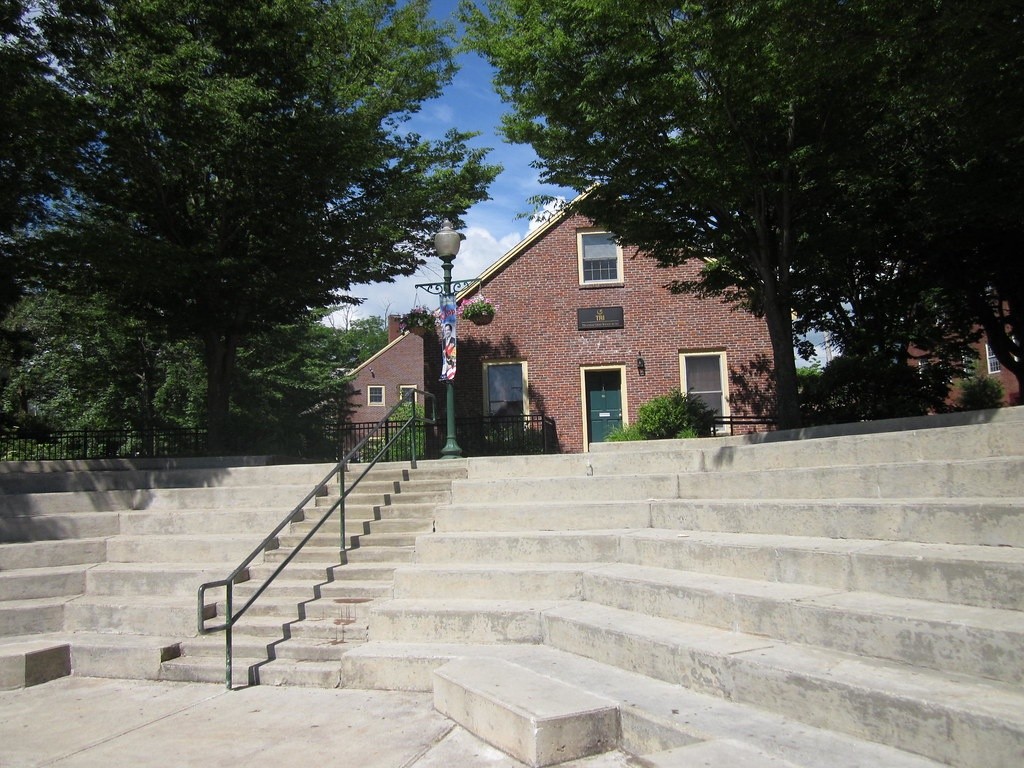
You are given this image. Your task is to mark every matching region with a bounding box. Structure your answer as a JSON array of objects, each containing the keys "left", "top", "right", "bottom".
[
  {"left": 413, "top": 326, "right": 426, "bottom": 335},
  {"left": 472, "top": 314, "right": 493, "bottom": 325}
]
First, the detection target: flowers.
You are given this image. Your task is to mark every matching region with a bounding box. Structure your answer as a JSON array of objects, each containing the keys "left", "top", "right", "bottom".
[
  {"left": 456, "top": 293, "right": 502, "bottom": 317},
  {"left": 398, "top": 306, "right": 442, "bottom": 330}
]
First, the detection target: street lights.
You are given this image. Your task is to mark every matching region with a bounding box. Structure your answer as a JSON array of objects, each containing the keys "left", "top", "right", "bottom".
[{"left": 415, "top": 219, "right": 481, "bottom": 461}]
